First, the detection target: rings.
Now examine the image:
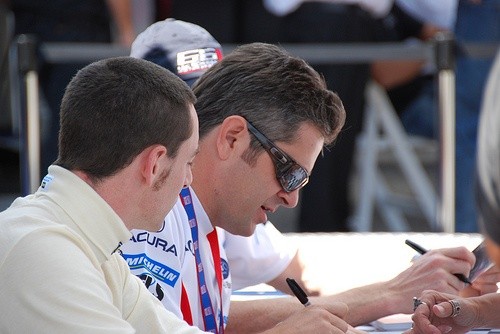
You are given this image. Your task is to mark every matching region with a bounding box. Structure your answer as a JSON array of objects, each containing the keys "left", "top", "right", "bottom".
[
  {"left": 413, "top": 297, "right": 429, "bottom": 312},
  {"left": 449, "top": 300, "right": 461, "bottom": 318}
]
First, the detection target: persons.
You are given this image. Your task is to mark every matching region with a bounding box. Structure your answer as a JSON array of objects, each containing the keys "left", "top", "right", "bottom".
[
  {"left": 0, "top": 0, "right": 460, "bottom": 233},
  {"left": 129, "top": 16, "right": 500, "bottom": 333},
  {"left": 412, "top": 50, "right": 500, "bottom": 334},
  {"left": 118, "top": 42, "right": 369, "bottom": 334},
  {"left": 0, "top": 56, "right": 351, "bottom": 334}
]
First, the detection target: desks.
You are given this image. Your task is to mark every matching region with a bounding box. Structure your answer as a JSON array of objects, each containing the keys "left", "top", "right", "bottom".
[{"left": 230, "top": 232, "right": 500, "bottom": 334}]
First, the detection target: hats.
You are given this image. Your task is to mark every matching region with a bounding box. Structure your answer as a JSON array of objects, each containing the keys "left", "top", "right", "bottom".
[{"left": 130, "top": 18, "right": 223, "bottom": 79}]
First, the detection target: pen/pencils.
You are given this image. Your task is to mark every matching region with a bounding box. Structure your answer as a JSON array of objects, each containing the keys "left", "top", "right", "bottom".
[
  {"left": 285, "top": 277, "right": 311, "bottom": 308},
  {"left": 405, "top": 239, "right": 472, "bottom": 285}
]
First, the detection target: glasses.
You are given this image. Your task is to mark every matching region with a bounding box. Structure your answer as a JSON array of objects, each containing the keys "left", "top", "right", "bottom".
[{"left": 218, "top": 114, "right": 310, "bottom": 193}]
